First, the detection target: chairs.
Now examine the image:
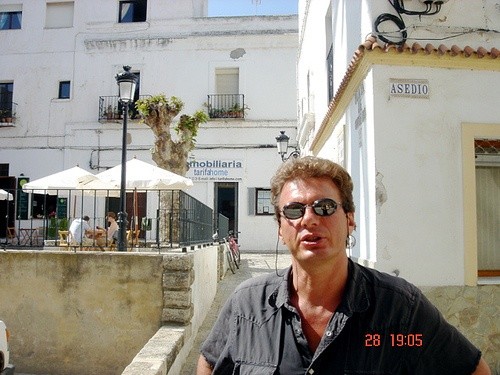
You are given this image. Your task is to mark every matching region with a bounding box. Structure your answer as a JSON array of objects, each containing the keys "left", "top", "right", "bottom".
[{"left": 8, "top": 226, "right": 140, "bottom": 251}]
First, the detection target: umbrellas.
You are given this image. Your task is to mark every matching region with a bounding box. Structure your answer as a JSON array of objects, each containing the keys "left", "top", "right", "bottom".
[
  {"left": 22, "top": 163, "right": 121, "bottom": 219},
  {"left": 95, "top": 155, "right": 194, "bottom": 244},
  {"left": 0, "top": 189, "right": 13, "bottom": 200}
]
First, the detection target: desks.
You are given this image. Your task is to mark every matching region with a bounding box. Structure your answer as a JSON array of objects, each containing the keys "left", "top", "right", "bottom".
[
  {"left": 84, "top": 230, "right": 105, "bottom": 251},
  {"left": 19, "top": 228, "right": 43, "bottom": 244}
]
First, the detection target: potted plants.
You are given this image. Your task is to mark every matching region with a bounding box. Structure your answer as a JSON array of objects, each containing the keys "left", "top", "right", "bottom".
[
  {"left": 0, "top": 110, "right": 12, "bottom": 122},
  {"left": 205, "top": 101, "right": 251, "bottom": 119}
]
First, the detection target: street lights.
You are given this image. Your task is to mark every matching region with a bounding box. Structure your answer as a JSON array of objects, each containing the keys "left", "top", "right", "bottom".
[{"left": 114, "top": 64, "right": 142, "bottom": 252}]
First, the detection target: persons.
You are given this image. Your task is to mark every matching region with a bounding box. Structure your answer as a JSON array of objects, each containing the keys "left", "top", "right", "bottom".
[
  {"left": 197, "top": 156, "right": 492, "bottom": 375},
  {"left": 67, "top": 216, "right": 96, "bottom": 245},
  {"left": 97, "top": 212, "right": 118, "bottom": 251},
  {"left": 320, "top": 204, "right": 330, "bottom": 215},
  {"left": 49, "top": 206, "right": 56, "bottom": 216},
  {"left": 0, "top": 320, "right": 10, "bottom": 371}
]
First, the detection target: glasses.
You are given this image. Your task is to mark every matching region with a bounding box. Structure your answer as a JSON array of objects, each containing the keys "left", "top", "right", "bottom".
[{"left": 281, "top": 198, "right": 339, "bottom": 219}]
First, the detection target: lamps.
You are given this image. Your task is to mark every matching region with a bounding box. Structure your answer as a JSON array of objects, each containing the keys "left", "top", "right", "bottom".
[{"left": 275, "top": 131, "right": 300, "bottom": 162}]
